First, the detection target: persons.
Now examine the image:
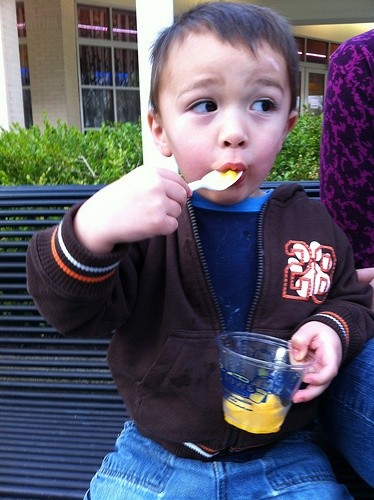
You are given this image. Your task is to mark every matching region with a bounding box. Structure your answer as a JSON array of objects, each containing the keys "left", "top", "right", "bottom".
[{"left": 25, "top": 0, "right": 374, "bottom": 500}]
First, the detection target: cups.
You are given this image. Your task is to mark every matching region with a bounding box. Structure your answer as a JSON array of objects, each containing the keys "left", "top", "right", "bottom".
[{"left": 213, "top": 331, "right": 317, "bottom": 434}]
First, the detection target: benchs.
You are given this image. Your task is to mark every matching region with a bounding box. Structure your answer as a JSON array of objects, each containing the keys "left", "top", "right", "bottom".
[{"left": 0, "top": 179, "right": 321, "bottom": 500}]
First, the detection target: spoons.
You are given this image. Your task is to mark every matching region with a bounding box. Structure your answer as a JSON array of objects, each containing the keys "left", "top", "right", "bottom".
[{"left": 188, "top": 169, "right": 244, "bottom": 192}]
